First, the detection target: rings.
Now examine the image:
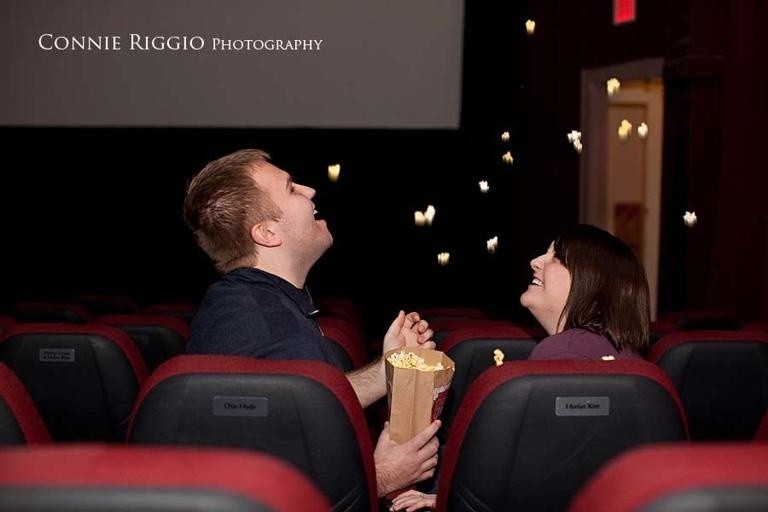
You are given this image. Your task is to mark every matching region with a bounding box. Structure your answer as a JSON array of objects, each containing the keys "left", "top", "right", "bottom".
[{"left": 418, "top": 494, "right": 423, "bottom": 497}]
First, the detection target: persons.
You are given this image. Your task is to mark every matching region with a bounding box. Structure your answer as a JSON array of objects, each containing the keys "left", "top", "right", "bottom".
[
  {"left": 185, "top": 150, "right": 441, "bottom": 499},
  {"left": 389, "top": 225, "right": 650, "bottom": 511}
]
